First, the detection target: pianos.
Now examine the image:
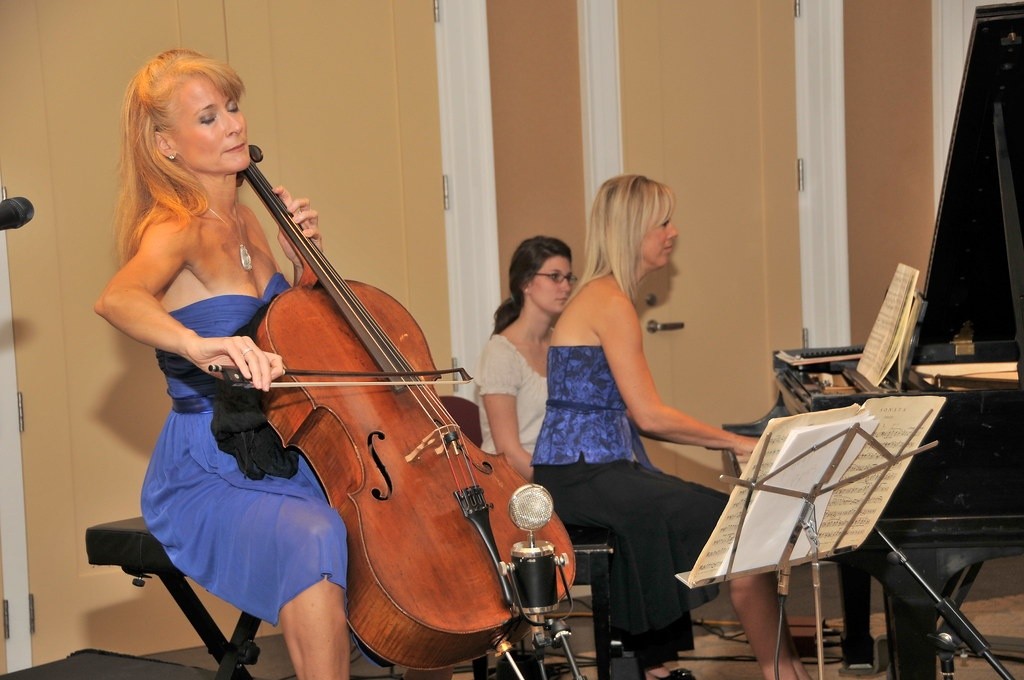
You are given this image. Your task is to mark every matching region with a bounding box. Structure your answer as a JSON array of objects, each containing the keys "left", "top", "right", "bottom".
[{"left": 718, "top": 0, "right": 1024, "bottom": 680}]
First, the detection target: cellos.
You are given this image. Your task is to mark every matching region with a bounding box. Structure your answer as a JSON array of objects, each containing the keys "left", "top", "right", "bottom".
[{"left": 208, "top": 141, "right": 580, "bottom": 672}]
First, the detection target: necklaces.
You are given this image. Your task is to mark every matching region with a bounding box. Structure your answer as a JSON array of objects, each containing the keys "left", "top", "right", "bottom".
[{"left": 209, "top": 203, "right": 252, "bottom": 272}]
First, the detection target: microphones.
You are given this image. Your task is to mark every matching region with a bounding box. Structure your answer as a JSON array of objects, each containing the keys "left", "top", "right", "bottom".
[{"left": 0, "top": 197, "right": 34, "bottom": 230}]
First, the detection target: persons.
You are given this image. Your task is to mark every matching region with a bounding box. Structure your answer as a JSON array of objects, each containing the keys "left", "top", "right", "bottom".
[
  {"left": 95, "top": 49, "right": 349, "bottom": 680},
  {"left": 530, "top": 175, "right": 811, "bottom": 680},
  {"left": 474, "top": 236, "right": 698, "bottom": 680}
]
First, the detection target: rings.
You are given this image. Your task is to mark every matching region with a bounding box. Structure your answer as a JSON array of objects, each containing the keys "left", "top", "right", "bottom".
[{"left": 243, "top": 349, "right": 254, "bottom": 357}]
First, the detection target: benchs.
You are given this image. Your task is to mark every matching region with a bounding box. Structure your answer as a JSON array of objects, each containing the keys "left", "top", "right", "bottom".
[
  {"left": 472, "top": 527, "right": 614, "bottom": 680},
  {"left": 85, "top": 516, "right": 262, "bottom": 680}
]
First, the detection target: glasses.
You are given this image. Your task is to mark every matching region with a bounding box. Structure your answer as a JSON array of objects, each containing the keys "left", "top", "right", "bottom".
[{"left": 534, "top": 272, "right": 577, "bottom": 284}]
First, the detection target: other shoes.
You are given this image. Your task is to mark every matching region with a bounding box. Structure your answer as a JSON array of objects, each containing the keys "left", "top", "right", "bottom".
[{"left": 657, "top": 668, "right": 696, "bottom": 680}]
